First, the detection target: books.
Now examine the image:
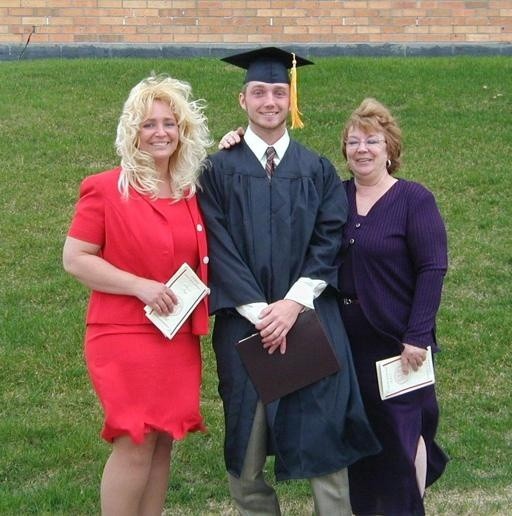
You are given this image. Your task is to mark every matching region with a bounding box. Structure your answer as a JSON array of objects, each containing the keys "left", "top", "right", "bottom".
[
  {"left": 143, "top": 262, "right": 210, "bottom": 340},
  {"left": 375, "top": 346, "right": 436, "bottom": 402},
  {"left": 235, "top": 309, "right": 340, "bottom": 407}
]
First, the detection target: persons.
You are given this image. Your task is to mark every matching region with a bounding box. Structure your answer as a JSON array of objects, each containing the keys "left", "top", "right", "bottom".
[
  {"left": 219, "top": 94, "right": 451, "bottom": 515},
  {"left": 194, "top": 46, "right": 383, "bottom": 516},
  {"left": 60, "top": 73, "right": 209, "bottom": 516}
]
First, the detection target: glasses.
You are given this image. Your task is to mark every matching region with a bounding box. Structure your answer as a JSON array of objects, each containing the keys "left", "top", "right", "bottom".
[{"left": 342, "top": 135, "right": 388, "bottom": 149}]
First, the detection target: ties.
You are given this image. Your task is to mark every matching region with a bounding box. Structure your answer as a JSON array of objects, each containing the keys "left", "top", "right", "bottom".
[{"left": 264, "top": 146, "right": 279, "bottom": 179}]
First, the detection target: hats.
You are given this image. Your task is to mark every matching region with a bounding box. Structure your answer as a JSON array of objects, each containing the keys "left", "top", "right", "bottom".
[{"left": 220, "top": 46, "right": 315, "bottom": 84}]
